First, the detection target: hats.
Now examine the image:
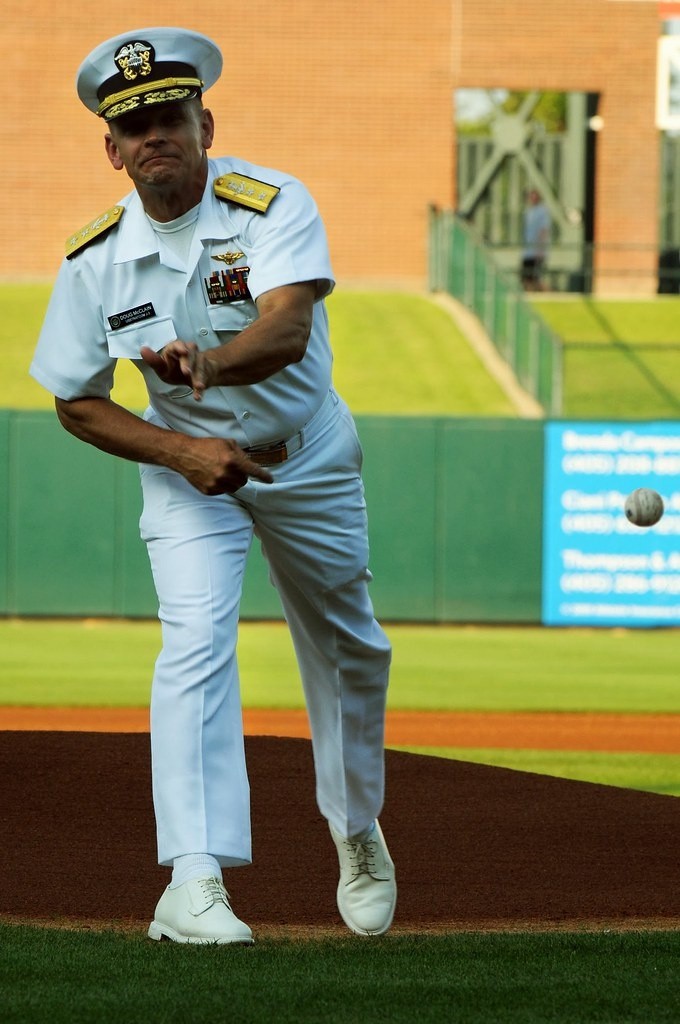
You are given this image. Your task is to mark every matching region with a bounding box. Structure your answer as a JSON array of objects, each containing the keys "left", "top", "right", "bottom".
[{"left": 76, "top": 28, "right": 223, "bottom": 123}]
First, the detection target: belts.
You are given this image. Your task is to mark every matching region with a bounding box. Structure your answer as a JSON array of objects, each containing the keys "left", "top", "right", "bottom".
[{"left": 247, "top": 393, "right": 335, "bottom": 466}]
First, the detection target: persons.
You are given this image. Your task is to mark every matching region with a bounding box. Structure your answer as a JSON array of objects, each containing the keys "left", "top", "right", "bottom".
[
  {"left": 521, "top": 185, "right": 553, "bottom": 292},
  {"left": 29, "top": 26, "right": 397, "bottom": 944}
]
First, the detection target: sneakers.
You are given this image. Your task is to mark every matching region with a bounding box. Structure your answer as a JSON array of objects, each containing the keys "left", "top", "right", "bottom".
[
  {"left": 147, "top": 876, "right": 255, "bottom": 945},
  {"left": 328, "top": 816, "right": 398, "bottom": 935}
]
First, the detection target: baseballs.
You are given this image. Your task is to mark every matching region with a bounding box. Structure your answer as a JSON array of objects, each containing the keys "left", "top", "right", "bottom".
[{"left": 624, "top": 487, "right": 664, "bottom": 527}]
